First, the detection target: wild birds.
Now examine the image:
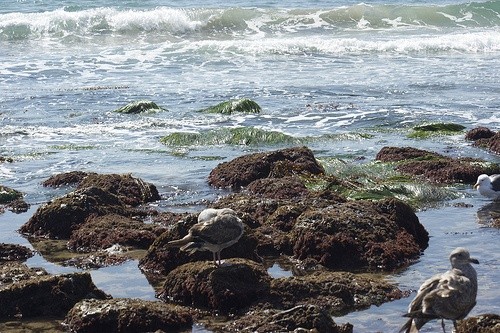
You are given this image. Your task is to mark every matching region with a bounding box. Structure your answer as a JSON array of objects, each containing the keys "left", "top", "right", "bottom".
[
  {"left": 167, "top": 208, "right": 243, "bottom": 269},
  {"left": 397, "top": 246, "right": 480, "bottom": 333},
  {"left": 474, "top": 174, "right": 500, "bottom": 199}
]
